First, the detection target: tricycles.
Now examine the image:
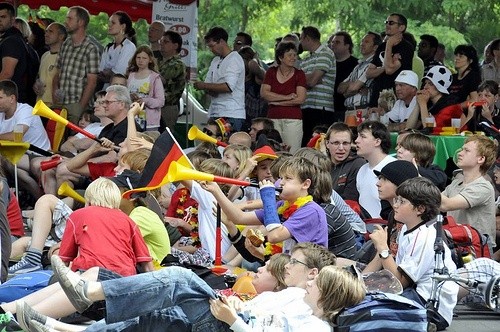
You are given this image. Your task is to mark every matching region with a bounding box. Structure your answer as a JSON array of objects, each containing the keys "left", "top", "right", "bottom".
[{"left": 425, "top": 212, "right": 500, "bottom": 332}]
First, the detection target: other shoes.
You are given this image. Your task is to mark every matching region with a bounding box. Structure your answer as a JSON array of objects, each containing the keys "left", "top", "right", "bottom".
[{"left": 47, "top": 244, "right": 61, "bottom": 261}]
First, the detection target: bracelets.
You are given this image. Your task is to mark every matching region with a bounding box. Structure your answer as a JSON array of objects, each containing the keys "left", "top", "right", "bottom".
[{"left": 217, "top": 57, "right": 224, "bottom": 69}]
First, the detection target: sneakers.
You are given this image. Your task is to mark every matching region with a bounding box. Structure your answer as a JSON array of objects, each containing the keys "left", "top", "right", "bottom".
[
  {"left": 50, "top": 254, "right": 93, "bottom": 314},
  {"left": 29, "top": 319, "right": 50, "bottom": 332},
  {"left": 7, "top": 257, "right": 43, "bottom": 275},
  {"left": 16, "top": 299, "right": 47, "bottom": 332}
]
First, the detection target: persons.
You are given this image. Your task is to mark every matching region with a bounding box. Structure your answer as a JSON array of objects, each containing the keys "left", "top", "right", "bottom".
[{"left": 0, "top": 3, "right": 500, "bottom": 332}]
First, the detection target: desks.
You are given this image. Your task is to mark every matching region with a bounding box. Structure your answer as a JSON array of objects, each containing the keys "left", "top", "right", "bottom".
[{"left": 386, "top": 130, "right": 492, "bottom": 182}]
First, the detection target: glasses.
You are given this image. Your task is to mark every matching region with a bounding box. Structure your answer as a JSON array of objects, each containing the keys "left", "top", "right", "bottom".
[
  {"left": 233, "top": 40, "right": 242, "bottom": 46},
  {"left": 393, "top": 197, "right": 411, "bottom": 204},
  {"left": 102, "top": 100, "right": 118, "bottom": 106},
  {"left": 288, "top": 258, "right": 309, "bottom": 267},
  {"left": 329, "top": 140, "right": 351, "bottom": 147},
  {"left": 384, "top": 20, "right": 404, "bottom": 25},
  {"left": 93, "top": 101, "right": 103, "bottom": 105},
  {"left": 158, "top": 38, "right": 172, "bottom": 46},
  {"left": 203, "top": 127, "right": 216, "bottom": 136}
]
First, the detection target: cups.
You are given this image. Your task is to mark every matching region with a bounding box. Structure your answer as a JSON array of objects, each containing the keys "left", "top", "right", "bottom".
[
  {"left": 379, "top": 115, "right": 390, "bottom": 126},
  {"left": 425, "top": 118, "right": 435, "bottom": 127},
  {"left": 450, "top": 118, "right": 461, "bottom": 133}
]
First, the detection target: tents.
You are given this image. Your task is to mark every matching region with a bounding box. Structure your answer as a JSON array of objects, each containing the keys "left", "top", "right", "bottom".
[{"left": 0, "top": 0, "right": 153, "bottom": 25}]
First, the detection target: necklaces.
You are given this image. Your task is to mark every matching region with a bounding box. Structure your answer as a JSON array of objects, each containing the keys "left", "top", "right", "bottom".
[
  {"left": 176, "top": 189, "right": 201, "bottom": 248},
  {"left": 315, "top": 46, "right": 318, "bottom": 51},
  {"left": 263, "top": 195, "right": 313, "bottom": 263},
  {"left": 280, "top": 67, "right": 291, "bottom": 79}
]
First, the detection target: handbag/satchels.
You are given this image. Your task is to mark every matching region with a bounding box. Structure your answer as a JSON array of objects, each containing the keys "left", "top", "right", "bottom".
[{"left": 0, "top": 270, "right": 54, "bottom": 304}]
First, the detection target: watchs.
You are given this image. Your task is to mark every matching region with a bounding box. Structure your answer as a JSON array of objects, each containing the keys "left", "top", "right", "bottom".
[{"left": 379, "top": 249, "right": 391, "bottom": 260}]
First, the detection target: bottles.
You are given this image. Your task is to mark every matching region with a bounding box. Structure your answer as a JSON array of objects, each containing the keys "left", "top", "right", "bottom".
[
  {"left": 368, "top": 111, "right": 378, "bottom": 121},
  {"left": 41, "top": 156, "right": 63, "bottom": 171}
]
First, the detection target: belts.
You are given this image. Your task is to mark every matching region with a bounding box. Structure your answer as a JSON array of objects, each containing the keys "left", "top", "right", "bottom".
[{"left": 347, "top": 104, "right": 368, "bottom": 110}]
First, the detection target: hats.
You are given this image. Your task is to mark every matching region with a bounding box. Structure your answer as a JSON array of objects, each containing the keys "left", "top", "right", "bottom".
[
  {"left": 248, "top": 133, "right": 279, "bottom": 179},
  {"left": 373, "top": 161, "right": 417, "bottom": 186},
  {"left": 99, "top": 169, "right": 146, "bottom": 198},
  {"left": 394, "top": 70, "right": 419, "bottom": 89},
  {"left": 214, "top": 117, "right": 230, "bottom": 141},
  {"left": 421, "top": 65, "right": 453, "bottom": 94}
]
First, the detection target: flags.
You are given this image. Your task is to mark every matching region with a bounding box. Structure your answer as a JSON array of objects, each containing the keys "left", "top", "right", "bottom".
[{"left": 121, "top": 130, "right": 195, "bottom": 202}]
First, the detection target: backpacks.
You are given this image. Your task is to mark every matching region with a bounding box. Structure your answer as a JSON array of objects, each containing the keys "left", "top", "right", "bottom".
[
  {"left": 329, "top": 288, "right": 428, "bottom": 332},
  {"left": 441, "top": 215, "right": 494, "bottom": 269},
  {"left": 180, "top": 262, "right": 236, "bottom": 289}
]
